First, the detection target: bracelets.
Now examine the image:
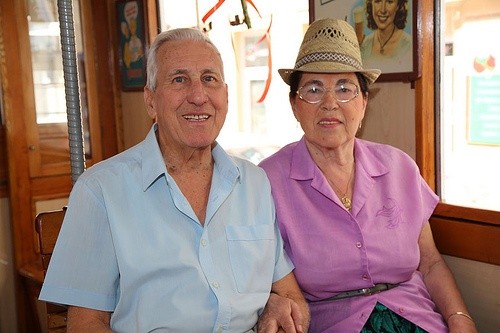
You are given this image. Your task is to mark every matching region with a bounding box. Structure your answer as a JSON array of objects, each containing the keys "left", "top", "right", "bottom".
[{"left": 446, "top": 311, "right": 472, "bottom": 320}]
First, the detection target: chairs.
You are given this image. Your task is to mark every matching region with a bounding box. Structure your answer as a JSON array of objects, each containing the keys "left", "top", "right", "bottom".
[{"left": 34, "top": 206, "right": 69, "bottom": 333}]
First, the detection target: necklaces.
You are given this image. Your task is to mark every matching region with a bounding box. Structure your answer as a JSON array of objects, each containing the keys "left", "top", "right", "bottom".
[{"left": 319, "top": 163, "right": 355, "bottom": 209}]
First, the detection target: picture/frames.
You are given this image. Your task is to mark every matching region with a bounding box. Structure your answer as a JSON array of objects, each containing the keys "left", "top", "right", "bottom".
[
  {"left": 116, "top": 0, "right": 149, "bottom": 93},
  {"left": 309, "top": 0, "right": 422, "bottom": 82}
]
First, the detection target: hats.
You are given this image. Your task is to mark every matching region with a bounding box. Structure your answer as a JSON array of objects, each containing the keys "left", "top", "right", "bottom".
[{"left": 277, "top": 17, "right": 382, "bottom": 89}]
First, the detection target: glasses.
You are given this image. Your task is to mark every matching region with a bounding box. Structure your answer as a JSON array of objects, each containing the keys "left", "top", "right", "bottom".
[{"left": 296, "top": 80, "right": 359, "bottom": 104}]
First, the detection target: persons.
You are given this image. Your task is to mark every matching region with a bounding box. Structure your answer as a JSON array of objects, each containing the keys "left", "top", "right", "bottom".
[
  {"left": 37, "top": 28, "right": 312, "bottom": 333},
  {"left": 255, "top": 18, "right": 477, "bottom": 333}
]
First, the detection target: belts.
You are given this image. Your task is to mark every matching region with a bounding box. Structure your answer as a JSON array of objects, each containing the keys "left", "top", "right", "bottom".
[{"left": 301, "top": 283, "right": 400, "bottom": 304}]
